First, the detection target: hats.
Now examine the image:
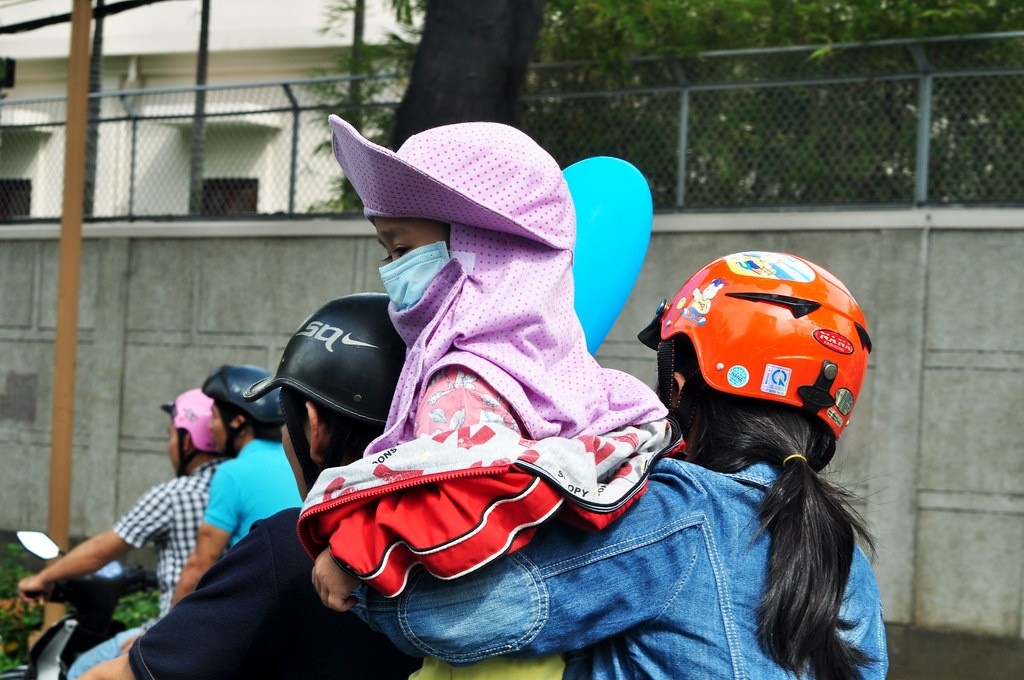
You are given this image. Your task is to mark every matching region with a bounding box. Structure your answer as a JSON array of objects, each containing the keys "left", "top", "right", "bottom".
[{"left": 327, "top": 112, "right": 574, "bottom": 252}]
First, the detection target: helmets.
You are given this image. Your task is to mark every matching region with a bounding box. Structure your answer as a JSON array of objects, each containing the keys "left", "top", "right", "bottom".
[
  {"left": 202, "top": 363, "right": 284, "bottom": 426},
  {"left": 245, "top": 292, "right": 392, "bottom": 422},
  {"left": 639, "top": 249, "right": 870, "bottom": 440},
  {"left": 163, "top": 389, "right": 223, "bottom": 453}
]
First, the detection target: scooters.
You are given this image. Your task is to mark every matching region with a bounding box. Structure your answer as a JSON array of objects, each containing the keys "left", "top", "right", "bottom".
[{"left": 17, "top": 529, "right": 167, "bottom": 680}]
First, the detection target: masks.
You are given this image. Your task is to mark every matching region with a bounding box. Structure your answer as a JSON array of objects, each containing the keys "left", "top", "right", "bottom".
[{"left": 379, "top": 242, "right": 451, "bottom": 312}]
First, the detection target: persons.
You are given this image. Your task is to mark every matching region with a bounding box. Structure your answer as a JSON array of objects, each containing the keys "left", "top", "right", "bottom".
[
  {"left": 73, "top": 292, "right": 427, "bottom": 680},
  {"left": 308, "top": 247, "right": 892, "bottom": 678},
  {"left": 322, "top": 105, "right": 673, "bottom": 678},
  {"left": 171, "top": 361, "right": 304, "bottom": 612},
  {"left": 16, "top": 387, "right": 242, "bottom": 679}
]
